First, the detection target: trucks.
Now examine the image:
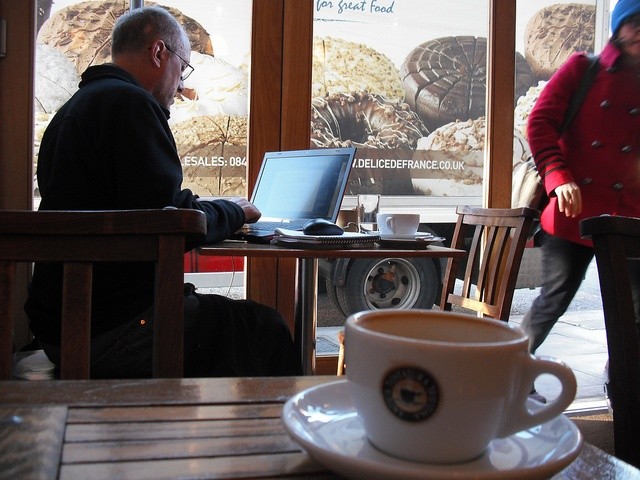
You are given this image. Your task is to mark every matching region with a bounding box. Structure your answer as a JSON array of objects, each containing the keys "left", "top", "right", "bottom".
[{"left": 35, "top": 0, "right": 611, "bottom": 321}]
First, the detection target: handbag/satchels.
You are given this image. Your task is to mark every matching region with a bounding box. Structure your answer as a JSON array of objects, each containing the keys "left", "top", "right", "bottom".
[{"left": 510, "top": 154, "right": 548, "bottom": 237}]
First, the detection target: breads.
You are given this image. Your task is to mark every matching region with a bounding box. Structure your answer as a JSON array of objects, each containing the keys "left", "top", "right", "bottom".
[
  {"left": 399, "top": 35, "right": 534, "bottom": 129},
  {"left": 169, "top": 114, "right": 250, "bottom": 203},
  {"left": 409, "top": 117, "right": 530, "bottom": 199},
  {"left": 514, "top": 80, "right": 550, "bottom": 145},
  {"left": 36, "top": 0, "right": 215, "bottom": 77},
  {"left": 522, "top": 2, "right": 595, "bottom": 81},
  {"left": 35, "top": 42, "right": 87, "bottom": 193},
  {"left": 312, "top": 34, "right": 403, "bottom": 104}
]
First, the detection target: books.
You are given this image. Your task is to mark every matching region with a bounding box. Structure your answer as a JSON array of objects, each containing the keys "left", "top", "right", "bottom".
[{"left": 274, "top": 229, "right": 381, "bottom": 251}]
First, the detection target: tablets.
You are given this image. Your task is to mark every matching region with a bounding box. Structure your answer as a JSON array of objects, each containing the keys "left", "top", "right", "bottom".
[{"left": 221, "top": 146, "right": 358, "bottom": 246}]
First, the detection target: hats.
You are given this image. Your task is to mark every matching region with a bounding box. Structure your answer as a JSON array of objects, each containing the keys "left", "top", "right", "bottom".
[{"left": 611, "top": 0, "right": 640, "bottom": 36}]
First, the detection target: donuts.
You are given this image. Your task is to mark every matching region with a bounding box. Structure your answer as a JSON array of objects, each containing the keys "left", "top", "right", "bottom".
[
  {"left": 164, "top": 49, "right": 248, "bottom": 124},
  {"left": 312, "top": 82, "right": 423, "bottom": 194}
]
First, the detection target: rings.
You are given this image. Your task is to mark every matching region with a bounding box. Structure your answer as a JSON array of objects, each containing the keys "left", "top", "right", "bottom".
[{"left": 566, "top": 199, "right": 571, "bottom": 204}]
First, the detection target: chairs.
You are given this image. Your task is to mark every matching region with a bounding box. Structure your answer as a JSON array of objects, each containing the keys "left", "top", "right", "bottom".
[
  {"left": 580, "top": 214, "right": 639, "bottom": 469},
  {"left": 337, "top": 205, "right": 538, "bottom": 375},
  {"left": 0, "top": 205, "right": 208, "bottom": 377}
]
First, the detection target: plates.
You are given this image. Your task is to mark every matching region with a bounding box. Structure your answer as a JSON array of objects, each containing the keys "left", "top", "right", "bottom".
[
  {"left": 372, "top": 232, "right": 431, "bottom": 239},
  {"left": 280, "top": 379, "right": 584, "bottom": 479}
]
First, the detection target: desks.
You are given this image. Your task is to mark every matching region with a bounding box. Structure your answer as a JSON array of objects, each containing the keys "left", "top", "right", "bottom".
[{"left": 198, "top": 235, "right": 469, "bottom": 373}]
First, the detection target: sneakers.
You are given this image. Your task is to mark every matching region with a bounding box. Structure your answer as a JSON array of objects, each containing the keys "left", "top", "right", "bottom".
[{"left": 529, "top": 389, "right": 547, "bottom": 403}]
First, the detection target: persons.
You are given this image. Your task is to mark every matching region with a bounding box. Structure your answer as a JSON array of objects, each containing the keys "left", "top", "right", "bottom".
[
  {"left": 520, "top": 0, "right": 640, "bottom": 403},
  {"left": 24, "top": 6, "right": 304, "bottom": 380}
]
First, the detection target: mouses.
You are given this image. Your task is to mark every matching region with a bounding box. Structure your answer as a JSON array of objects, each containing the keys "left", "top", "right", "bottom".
[{"left": 303, "top": 217, "right": 346, "bottom": 234}]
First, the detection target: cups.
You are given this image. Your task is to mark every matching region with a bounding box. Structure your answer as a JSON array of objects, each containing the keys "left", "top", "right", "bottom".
[
  {"left": 376, "top": 213, "right": 420, "bottom": 234},
  {"left": 357, "top": 193, "right": 381, "bottom": 230},
  {"left": 344, "top": 310, "right": 578, "bottom": 464}
]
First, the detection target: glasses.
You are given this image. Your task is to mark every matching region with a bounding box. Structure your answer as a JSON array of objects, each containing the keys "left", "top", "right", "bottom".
[{"left": 149, "top": 42, "right": 194, "bottom": 81}]
